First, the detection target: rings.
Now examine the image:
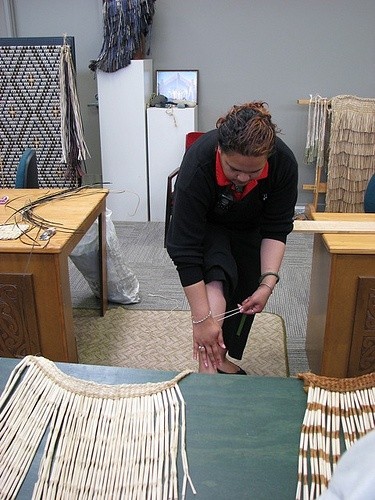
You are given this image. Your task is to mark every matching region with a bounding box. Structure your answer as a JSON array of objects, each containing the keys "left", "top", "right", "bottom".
[
  {"left": 199, "top": 345, "right": 204, "bottom": 350},
  {"left": 251, "top": 309, "right": 254, "bottom": 315}
]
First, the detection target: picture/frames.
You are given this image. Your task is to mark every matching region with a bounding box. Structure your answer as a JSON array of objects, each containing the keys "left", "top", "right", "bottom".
[{"left": 156, "top": 69, "right": 198, "bottom": 105}]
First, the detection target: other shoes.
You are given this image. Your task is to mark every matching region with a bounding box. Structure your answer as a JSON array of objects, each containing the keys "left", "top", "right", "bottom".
[{"left": 217, "top": 360, "right": 247, "bottom": 374}]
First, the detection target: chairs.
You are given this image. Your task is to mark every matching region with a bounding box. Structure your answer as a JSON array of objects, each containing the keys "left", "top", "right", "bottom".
[{"left": 15, "top": 149, "right": 39, "bottom": 189}]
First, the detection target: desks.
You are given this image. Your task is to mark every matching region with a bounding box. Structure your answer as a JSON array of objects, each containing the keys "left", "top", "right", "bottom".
[
  {"left": 305, "top": 212, "right": 375, "bottom": 378},
  {"left": 0, "top": 357, "right": 375, "bottom": 500},
  {"left": 0, "top": 188, "right": 110, "bottom": 363}
]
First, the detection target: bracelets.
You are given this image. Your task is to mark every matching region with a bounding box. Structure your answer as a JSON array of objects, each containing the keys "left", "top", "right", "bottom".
[
  {"left": 192, "top": 310, "right": 211, "bottom": 324},
  {"left": 258, "top": 271, "right": 280, "bottom": 284},
  {"left": 259, "top": 283, "right": 272, "bottom": 294}
]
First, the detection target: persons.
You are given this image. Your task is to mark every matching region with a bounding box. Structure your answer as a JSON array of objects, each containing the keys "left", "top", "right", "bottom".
[{"left": 163, "top": 101, "right": 299, "bottom": 374}]
